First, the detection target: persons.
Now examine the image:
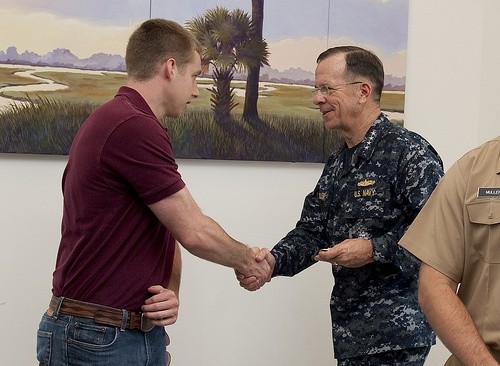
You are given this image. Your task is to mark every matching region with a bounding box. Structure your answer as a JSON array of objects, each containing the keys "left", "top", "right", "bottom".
[
  {"left": 37, "top": 18, "right": 271, "bottom": 365},
  {"left": 398, "top": 134, "right": 499, "bottom": 366},
  {"left": 236, "top": 47, "right": 444, "bottom": 366}
]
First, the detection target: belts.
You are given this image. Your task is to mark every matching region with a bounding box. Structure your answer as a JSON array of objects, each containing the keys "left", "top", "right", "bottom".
[{"left": 48, "top": 296, "right": 154, "bottom": 331}]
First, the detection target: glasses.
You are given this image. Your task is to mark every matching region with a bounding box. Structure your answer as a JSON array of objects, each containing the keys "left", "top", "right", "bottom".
[{"left": 311, "top": 81, "right": 364, "bottom": 98}]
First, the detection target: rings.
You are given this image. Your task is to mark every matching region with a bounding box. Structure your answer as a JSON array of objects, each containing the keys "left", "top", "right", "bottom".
[{"left": 333, "top": 260, "right": 339, "bottom": 266}]
